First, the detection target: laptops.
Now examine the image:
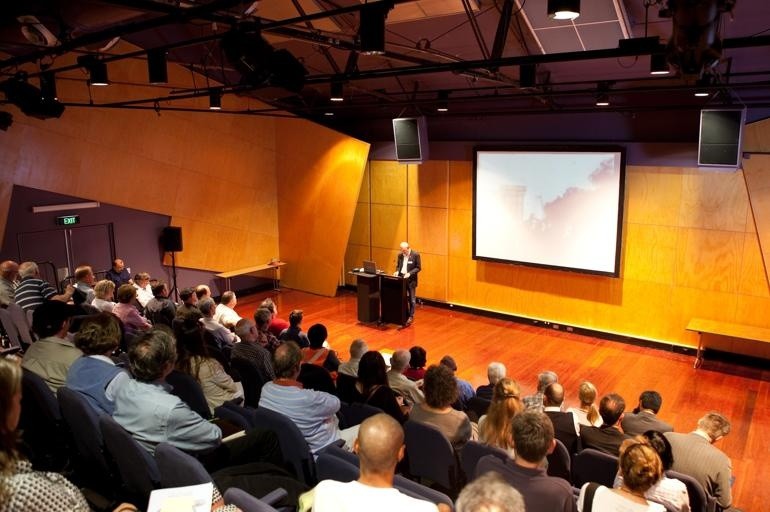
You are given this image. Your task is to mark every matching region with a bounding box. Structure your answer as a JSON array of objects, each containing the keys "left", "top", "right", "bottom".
[{"left": 362, "top": 260, "right": 380, "bottom": 274}]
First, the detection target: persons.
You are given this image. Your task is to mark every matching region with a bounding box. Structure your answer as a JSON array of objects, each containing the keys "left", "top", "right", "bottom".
[
  {"left": 1, "top": 258, "right": 743, "bottom": 510},
  {"left": 392, "top": 242, "right": 421, "bottom": 324}
]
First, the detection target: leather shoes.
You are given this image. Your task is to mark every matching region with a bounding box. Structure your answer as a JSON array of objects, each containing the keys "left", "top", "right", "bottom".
[{"left": 406, "top": 316, "right": 412, "bottom": 324}]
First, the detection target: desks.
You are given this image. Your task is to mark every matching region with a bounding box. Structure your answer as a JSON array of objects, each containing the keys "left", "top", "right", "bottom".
[
  {"left": 212, "top": 258, "right": 288, "bottom": 302},
  {"left": 680, "top": 318, "right": 769, "bottom": 369}
]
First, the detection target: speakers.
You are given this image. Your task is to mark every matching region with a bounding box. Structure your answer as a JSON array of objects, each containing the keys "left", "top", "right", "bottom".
[
  {"left": 392, "top": 116, "right": 430, "bottom": 164},
  {"left": 697, "top": 107, "right": 747, "bottom": 168},
  {"left": 162, "top": 226, "right": 182, "bottom": 251}
]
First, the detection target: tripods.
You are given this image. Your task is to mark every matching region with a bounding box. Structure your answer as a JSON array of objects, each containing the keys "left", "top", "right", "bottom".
[{"left": 165, "top": 252, "right": 186, "bottom": 303}]
